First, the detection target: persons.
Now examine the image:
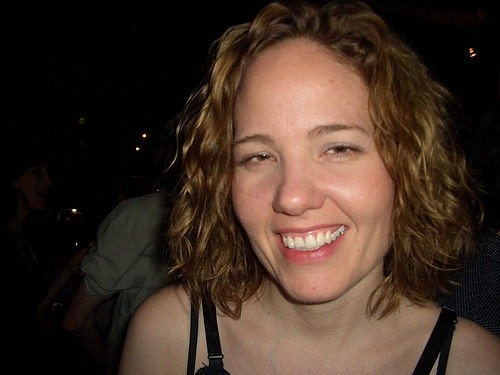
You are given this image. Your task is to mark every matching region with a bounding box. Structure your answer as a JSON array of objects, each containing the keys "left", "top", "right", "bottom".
[
  {"left": 0, "top": 132, "right": 197, "bottom": 375},
  {"left": 427, "top": 106, "right": 500, "bottom": 338},
  {"left": 119, "top": 0, "right": 500, "bottom": 375}
]
